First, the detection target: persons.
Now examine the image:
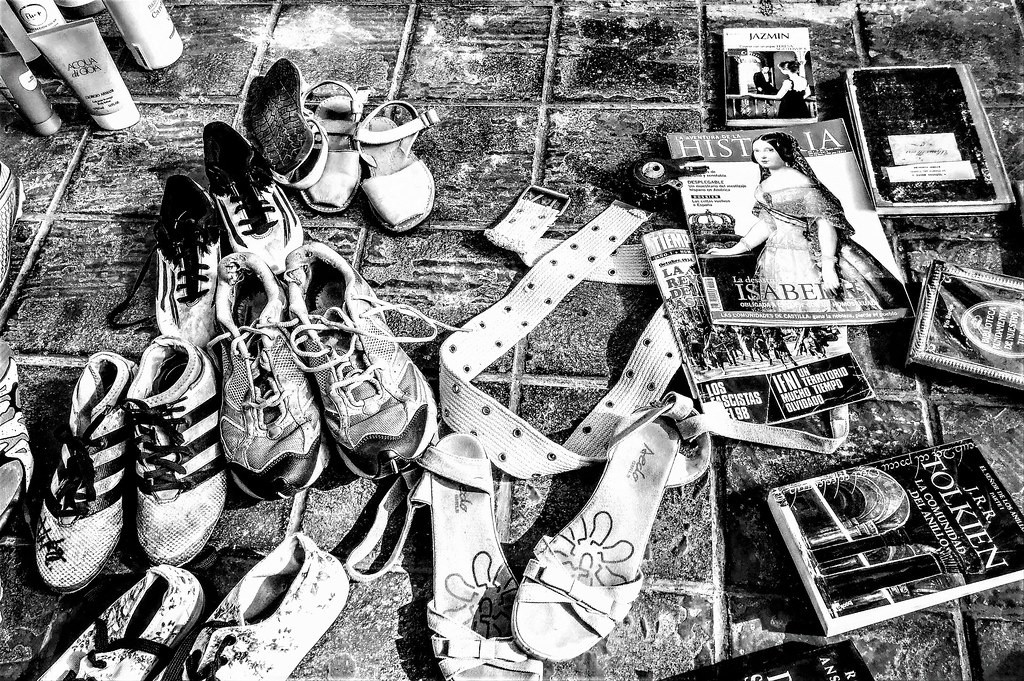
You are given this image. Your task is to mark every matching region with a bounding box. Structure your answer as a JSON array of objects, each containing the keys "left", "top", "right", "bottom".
[
  {"left": 702, "top": 134, "right": 905, "bottom": 311},
  {"left": 754, "top": 64, "right": 779, "bottom": 107},
  {"left": 742, "top": 61, "right": 812, "bottom": 118}
]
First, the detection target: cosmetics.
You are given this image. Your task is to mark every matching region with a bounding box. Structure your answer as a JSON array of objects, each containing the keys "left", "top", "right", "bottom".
[
  {"left": 28, "top": 18, "right": 141, "bottom": 130},
  {"left": 103, "top": 0, "right": 183, "bottom": 69},
  {"left": 52, "top": 0, "right": 107, "bottom": 19},
  {"left": 0, "top": 52, "right": 62, "bottom": 135},
  {"left": 9, "top": 1, "right": 68, "bottom": 75},
  {"left": 0, "top": 1, "right": 56, "bottom": 80}
]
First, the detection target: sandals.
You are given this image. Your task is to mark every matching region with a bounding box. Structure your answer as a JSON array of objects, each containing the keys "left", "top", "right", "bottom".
[
  {"left": 345, "top": 433, "right": 543, "bottom": 681},
  {"left": 512, "top": 392, "right": 850, "bottom": 665},
  {"left": 353, "top": 100, "right": 441, "bottom": 233},
  {"left": 295, "top": 80, "right": 362, "bottom": 213},
  {"left": 243, "top": 56, "right": 329, "bottom": 190}
]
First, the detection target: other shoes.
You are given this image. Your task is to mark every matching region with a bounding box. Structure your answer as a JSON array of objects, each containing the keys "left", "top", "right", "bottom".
[
  {"left": 0, "top": 339, "right": 35, "bottom": 502},
  {"left": 0, "top": 460, "right": 35, "bottom": 550}
]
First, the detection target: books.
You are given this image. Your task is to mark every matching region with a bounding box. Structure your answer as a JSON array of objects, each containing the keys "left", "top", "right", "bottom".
[
  {"left": 768, "top": 436, "right": 1023, "bottom": 635},
  {"left": 849, "top": 64, "right": 1015, "bottom": 215},
  {"left": 643, "top": 121, "right": 1024, "bottom": 454},
  {"left": 723, "top": 23, "right": 822, "bottom": 129},
  {"left": 658, "top": 642, "right": 874, "bottom": 681}
]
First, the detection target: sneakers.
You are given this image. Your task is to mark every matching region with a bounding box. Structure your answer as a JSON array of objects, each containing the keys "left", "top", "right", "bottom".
[
  {"left": 33, "top": 352, "right": 140, "bottom": 596},
  {"left": 205, "top": 250, "right": 328, "bottom": 502},
  {"left": 284, "top": 242, "right": 436, "bottom": 480},
  {"left": 36, "top": 565, "right": 206, "bottom": 681},
  {"left": 0, "top": 161, "right": 25, "bottom": 298},
  {"left": 105, "top": 175, "right": 223, "bottom": 349},
  {"left": 152, "top": 531, "right": 350, "bottom": 681},
  {"left": 202, "top": 120, "right": 303, "bottom": 274},
  {"left": 119, "top": 334, "right": 228, "bottom": 568}
]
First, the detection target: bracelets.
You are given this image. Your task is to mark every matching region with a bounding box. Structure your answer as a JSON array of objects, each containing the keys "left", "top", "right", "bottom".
[
  {"left": 740, "top": 238, "right": 751, "bottom": 251},
  {"left": 820, "top": 256, "right": 838, "bottom": 262}
]
